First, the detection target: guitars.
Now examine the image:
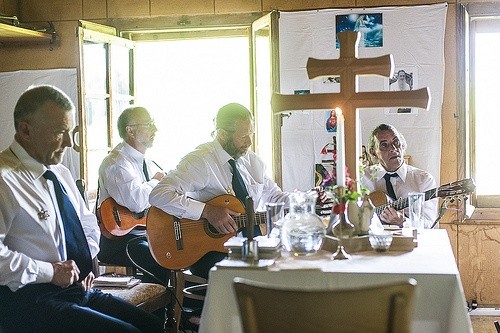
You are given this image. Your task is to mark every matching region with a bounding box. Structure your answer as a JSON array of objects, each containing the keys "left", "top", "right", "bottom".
[
  {"left": 144, "top": 194, "right": 332, "bottom": 271},
  {"left": 369, "top": 177, "right": 477, "bottom": 224},
  {"left": 95, "top": 195, "right": 147, "bottom": 242}
]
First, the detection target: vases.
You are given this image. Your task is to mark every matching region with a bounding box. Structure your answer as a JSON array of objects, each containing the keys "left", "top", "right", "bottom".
[{"left": 346, "top": 196, "right": 375, "bottom": 233}]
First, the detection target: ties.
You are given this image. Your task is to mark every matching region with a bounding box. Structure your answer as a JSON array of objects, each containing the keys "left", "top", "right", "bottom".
[
  {"left": 143, "top": 160, "right": 150, "bottom": 182},
  {"left": 8, "top": 145, "right": 93, "bottom": 284},
  {"left": 228, "top": 159, "right": 262, "bottom": 238},
  {"left": 383, "top": 173, "right": 410, "bottom": 218}
]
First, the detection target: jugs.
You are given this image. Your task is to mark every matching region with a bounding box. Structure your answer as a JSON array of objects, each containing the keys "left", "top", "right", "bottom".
[{"left": 281, "top": 191, "right": 326, "bottom": 257}]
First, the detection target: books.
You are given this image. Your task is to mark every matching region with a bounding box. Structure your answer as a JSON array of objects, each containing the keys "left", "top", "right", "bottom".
[
  {"left": 224, "top": 235, "right": 282, "bottom": 259},
  {"left": 387, "top": 230, "right": 420, "bottom": 251}
]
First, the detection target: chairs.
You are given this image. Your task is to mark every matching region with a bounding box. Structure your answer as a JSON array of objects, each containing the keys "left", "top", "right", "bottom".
[{"left": 74, "top": 179, "right": 178, "bottom": 333}]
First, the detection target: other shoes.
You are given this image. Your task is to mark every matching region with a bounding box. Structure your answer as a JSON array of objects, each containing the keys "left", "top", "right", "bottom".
[{"left": 166, "top": 311, "right": 199, "bottom": 330}]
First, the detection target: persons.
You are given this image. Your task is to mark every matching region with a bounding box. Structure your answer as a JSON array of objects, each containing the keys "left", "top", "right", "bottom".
[
  {"left": 149, "top": 103, "right": 327, "bottom": 333},
  {"left": 0, "top": 84, "right": 164, "bottom": 333},
  {"left": 359, "top": 123, "right": 439, "bottom": 229},
  {"left": 92, "top": 107, "right": 172, "bottom": 333}
]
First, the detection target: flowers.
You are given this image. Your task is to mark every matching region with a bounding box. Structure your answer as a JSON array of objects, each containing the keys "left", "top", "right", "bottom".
[{"left": 331, "top": 168, "right": 379, "bottom": 214}]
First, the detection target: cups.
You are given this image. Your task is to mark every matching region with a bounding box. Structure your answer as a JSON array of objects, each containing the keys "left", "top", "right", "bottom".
[
  {"left": 265, "top": 202, "right": 285, "bottom": 238},
  {"left": 408, "top": 192, "right": 425, "bottom": 234},
  {"left": 368, "top": 230, "right": 393, "bottom": 252}
]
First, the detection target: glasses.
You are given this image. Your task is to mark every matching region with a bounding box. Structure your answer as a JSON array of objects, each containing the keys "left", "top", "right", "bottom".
[
  {"left": 216, "top": 126, "right": 255, "bottom": 142},
  {"left": 372, "top": 139, "right": 402, "bottom": 152},
  {"left": 125, "top": 117, "right": 155, "bottom": 132}
]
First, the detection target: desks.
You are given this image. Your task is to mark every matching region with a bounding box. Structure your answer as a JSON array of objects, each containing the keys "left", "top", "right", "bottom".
[
  {"left": 233, "top": 278, "right": 417, "bottom": 332},
  {"left": 199, "top": 228, "right": 474, "bottom": 333}
]
